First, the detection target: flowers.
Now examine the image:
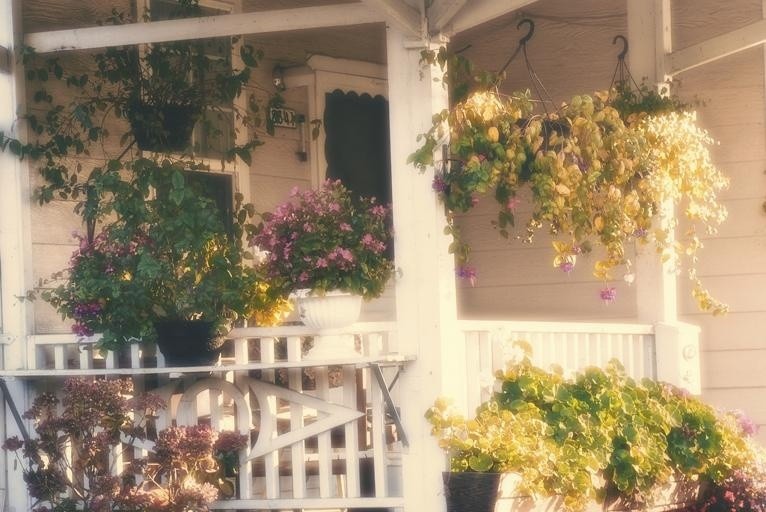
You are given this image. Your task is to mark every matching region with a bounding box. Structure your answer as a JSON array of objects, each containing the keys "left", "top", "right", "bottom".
[
  {"left": 64, "top": 227, "right": 156, "bottom": 334},
  {"left": 410, "top": 45, "right": 736, "bottom": 318},
  {"left": 249, "top": 177, "right": 396, "bottom": 299}
]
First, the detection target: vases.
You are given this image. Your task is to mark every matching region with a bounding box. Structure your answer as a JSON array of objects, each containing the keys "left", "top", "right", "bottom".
[
  {"left": 294, "top": 292, "right": 364, "bottom": 359},
  {"left": 443, "top": 471, "right": 500, "bottom": 512}
]
